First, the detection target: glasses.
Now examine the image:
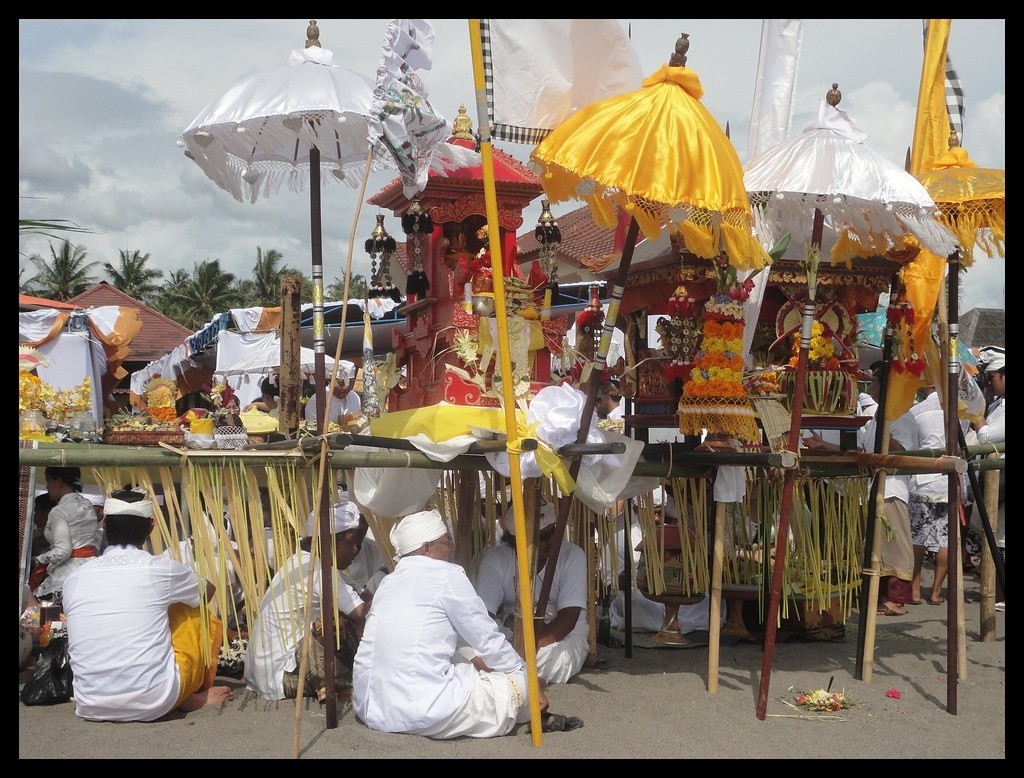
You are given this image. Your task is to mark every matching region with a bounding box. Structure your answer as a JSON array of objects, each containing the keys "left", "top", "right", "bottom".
[
  {"left": 432, "top": 539, "right": 451, "bottom": 547},
  {"left": 540, "top": 527, "right": 555, "bottom": 541}
]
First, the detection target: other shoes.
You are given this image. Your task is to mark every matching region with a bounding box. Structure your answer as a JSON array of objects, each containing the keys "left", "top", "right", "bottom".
[{"left": 995, "top": 601, "right": 1005, "bottom": 611}]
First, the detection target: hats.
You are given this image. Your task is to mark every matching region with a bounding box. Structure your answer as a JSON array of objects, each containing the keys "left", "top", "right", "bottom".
[
  {"left": 327, "top": 360, "right": 356, "bottom": 380},
  {"left": 103, "top": 489, "right": 153, "bottom": 518},
  {"left": 505, "top": 497, "right": 556, "bottom": 536},
  {"left": 338, "top": 490, "right": 363, "bottom": 514},
  {"left": 979, "top": 345, "right": 1005, "bottom": 373},
  {"left": 633, "top": 483, "right": 667, "bottom": 508},
  {"left": 196, "top": 510, "right": 232, "bottom": 550},
  {"left": 389, "top": 509, "right": 447, "bottom": 558},
  {"left": 307, "top": 501, "right": 359, "bottom": 537}
]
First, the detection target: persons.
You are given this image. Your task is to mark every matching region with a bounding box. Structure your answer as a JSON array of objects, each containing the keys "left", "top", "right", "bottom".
[
  {"left": 242, "top": 373, "right": 280, "bottom": 419},
  {"left": 244, "top": 484, "right": 365, "bottom": 701},
  {"left": 887, "top": 387, "right": 948, "bottom": 605},
  {"left": 304, "top": 359, "right": 362, "bottom": 433},
  {"left": 352, "top": 510, "right": 585, "bottom": 741},
  {"left": 600, "top": 379, "right": 626, "bottom": 434},
  {"left": 339, "top": 513, "right": 388, "bottom": 602},
  {"left": 785, "top": 344, "right": 999, "bottom": 455},
  {"left": 29, "top": 466, "right": 103, "bottom": 599},
  {"left": 212, "top": 392, "right": 244, "bottom": 430},
  {"left": 474, "top": 494, "right": 591, "bottom": 685},
  {"left": 802, "top": 360, "right": 915, "bottom": 616},
  {"left": 164, "top": 510, "right": 300, "bottom": 628},
  {"left": 964, "top": 358, "right": 1005, "bottom": 613},
  {"left": 596, "top": 484, "right": 728, "bottom": 634},
  {"left": 62, "top": 482, "right": 234, "bottom": 723}
]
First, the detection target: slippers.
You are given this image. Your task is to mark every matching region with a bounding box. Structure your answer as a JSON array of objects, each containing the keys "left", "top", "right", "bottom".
[
  {"left": 927, "top": 595, "right": 946, "bottom": 605},
  {"left": 877, "top": 601, "right": 905, "bottom": 615},
  {"left": 525, "top": 713, "right": 584, "bottom": 734},
  {"left": 905, "top": 601, "right": 921, "bottom": 604},
  {"left": 584, "top": 653, "right": 610, "bottom": 670}
]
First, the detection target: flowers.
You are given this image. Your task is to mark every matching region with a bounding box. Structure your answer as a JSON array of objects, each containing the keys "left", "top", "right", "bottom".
[{"left": 682, "top": 232, "right": 839, "bottom": 399}]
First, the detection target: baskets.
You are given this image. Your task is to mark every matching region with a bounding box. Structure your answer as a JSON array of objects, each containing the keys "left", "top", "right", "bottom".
[{"left": 103, "top": 431, "right": 185, "bottom": 446}]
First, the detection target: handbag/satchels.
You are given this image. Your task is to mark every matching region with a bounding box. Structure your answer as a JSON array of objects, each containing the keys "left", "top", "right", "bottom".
[{"left": 21, "top": 636, "right": 73, "bottom": 706}]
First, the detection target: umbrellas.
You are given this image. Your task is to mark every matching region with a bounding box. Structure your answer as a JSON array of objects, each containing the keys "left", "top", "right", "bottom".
[
  {"left": 175, "top": 19, "right": 484, "bottom": 730},
  {"left": 918, "top": 128, "right": 1005, "bottom": 717},
  {"left": 741, "top": 81, "right": 961, "bottom": 721},
  {"left": 529, "top": 31, "right": 776, "bottom": 656}
]
